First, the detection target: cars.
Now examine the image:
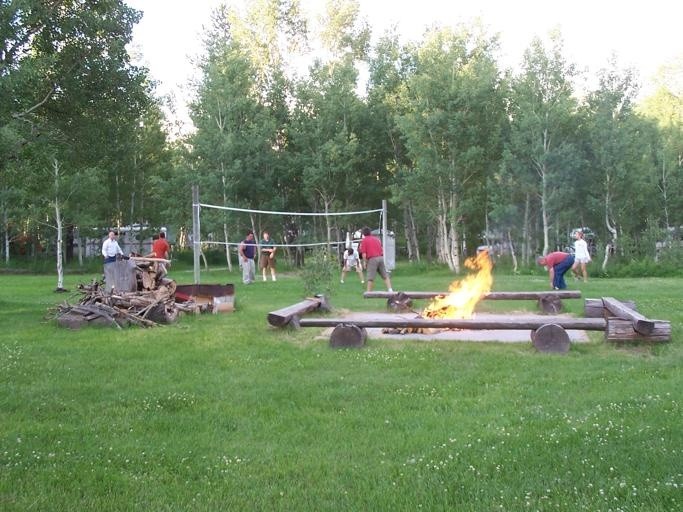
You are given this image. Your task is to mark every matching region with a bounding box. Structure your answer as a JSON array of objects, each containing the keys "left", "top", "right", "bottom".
[{"left": 570, "top": 227, "right": 595, "bottom": 240}]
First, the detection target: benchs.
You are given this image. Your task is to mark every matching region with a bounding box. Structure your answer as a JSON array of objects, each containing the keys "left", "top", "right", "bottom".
[
  {"left": 299, "top": 316, "right": 608, "bottom": 351},
  {"left": 585, "top": 297, "right": 672, "bottom": 343},
  {"left": 270, "top": 298, "right": 325, "bottom": 330},
  {"left": 363, "top": 291, "right": 582, "bottom": 312}
]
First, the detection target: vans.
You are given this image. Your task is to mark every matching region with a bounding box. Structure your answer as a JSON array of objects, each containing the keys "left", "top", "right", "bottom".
[{"left": 476, "top": 235, "right": 507, "bottom": 256}]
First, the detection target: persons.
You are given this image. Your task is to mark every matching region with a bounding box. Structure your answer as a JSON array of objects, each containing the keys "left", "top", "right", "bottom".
[
  {"left": 98, "top": 231, "right": 124, "bottom": 283},
  {"left": 150, "top": 231, "right": 172, "bottom": 283},
  {"left": 258, "top": 230, "right": 277, "bottom": 282},
  {"left": 238, "top": 230, "right": 256, "bottom": 285},
  {"left": 534, "top": 250, "right": 575, "bottom": 290},
  {"left": 571, "top": 230, "right": 592, "bottom": 282},
  {"left": 357, "top": 227, "right": 392, "bottom": 292},
  {"left": 338, "top": 248, "right": 365, "bottom": 284}
]
